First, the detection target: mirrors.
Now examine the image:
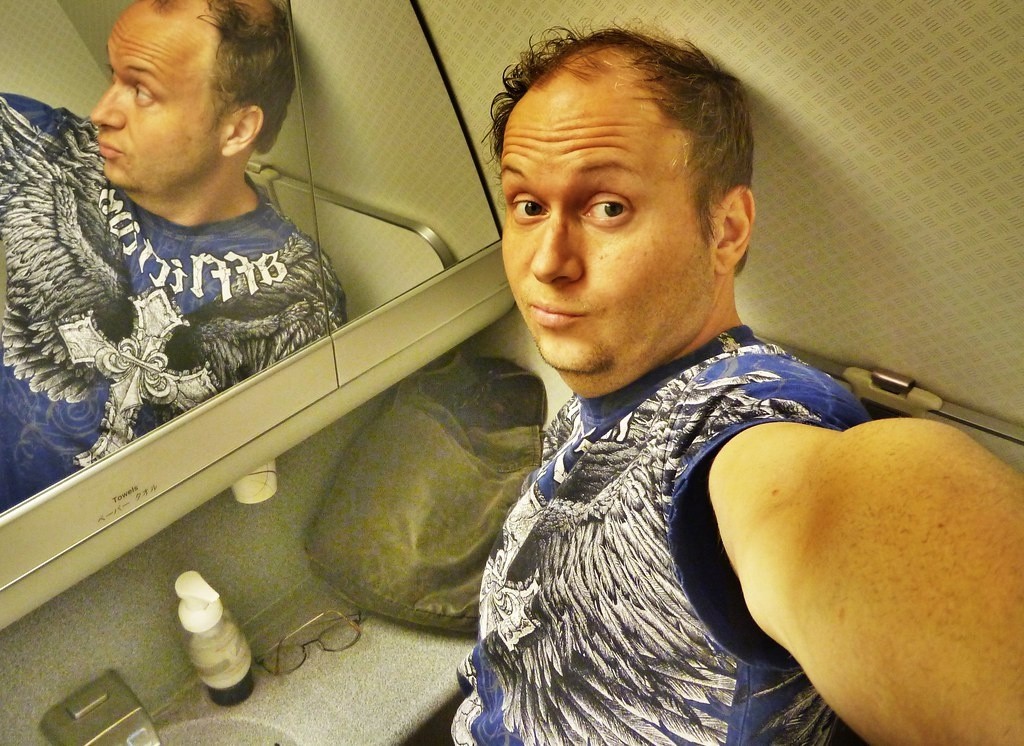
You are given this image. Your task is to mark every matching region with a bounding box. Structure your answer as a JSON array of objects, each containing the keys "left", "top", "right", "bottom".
[{"left": 0, "top": 0, "right": 518, "bottom": 634}]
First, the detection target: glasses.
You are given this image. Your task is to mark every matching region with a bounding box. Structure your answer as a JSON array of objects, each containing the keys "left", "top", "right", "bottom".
[{"left": 254, "top": 609, "right": 363, "bottom": 675}]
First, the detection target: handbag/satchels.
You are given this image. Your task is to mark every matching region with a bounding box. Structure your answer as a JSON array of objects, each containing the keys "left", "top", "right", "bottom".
[{"left": 299, "top": 351, "right": 551, "bottom": 633}]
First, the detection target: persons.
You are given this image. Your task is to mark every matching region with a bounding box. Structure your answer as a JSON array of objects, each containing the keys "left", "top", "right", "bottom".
[
  {"left": 0, "top": 1, "right": 348, "bottom": 516},
  {"left": 450, "top": 29, "right": 1023, "bottom": 746}
]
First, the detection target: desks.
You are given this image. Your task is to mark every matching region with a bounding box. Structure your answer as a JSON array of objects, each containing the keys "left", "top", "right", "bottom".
[{"left": 154, "top": 603, "right": 478, "bottom": 745}]
